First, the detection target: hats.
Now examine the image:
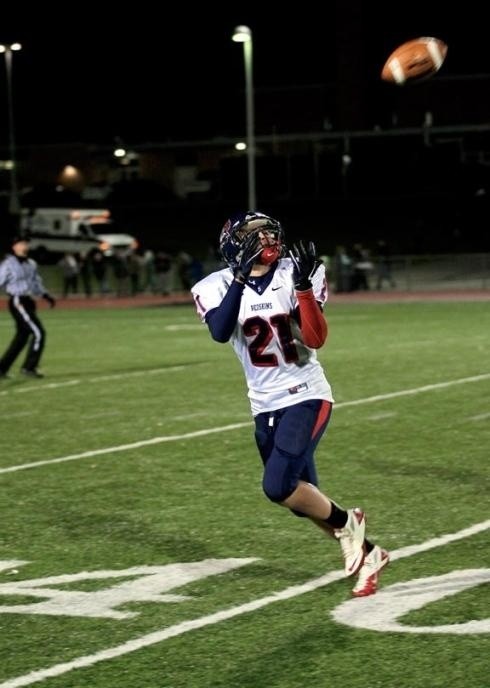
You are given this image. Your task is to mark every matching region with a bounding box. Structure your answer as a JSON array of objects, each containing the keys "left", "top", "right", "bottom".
[{"left": 11, "top": 235, "right": 31, "bottom": 245}]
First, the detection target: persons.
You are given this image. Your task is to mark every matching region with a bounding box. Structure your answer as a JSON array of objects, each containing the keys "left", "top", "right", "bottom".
[
  {"left": 189, "top": 211, "right": 389, "bottom": 597},
  {"left": 318, "top": 244, "right": 372, "bottom": 294},
  {"left": 59, "top": 243, "right": 229, "bottom": 298},
  {"left": 0, "top": 236, "right": 55, "bottom": 380}
]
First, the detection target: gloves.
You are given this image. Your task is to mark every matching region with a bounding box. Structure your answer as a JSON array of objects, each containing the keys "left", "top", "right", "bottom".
[
  {"left": 289, "top": 240, "right": 323, "bottom": 290},
  {"left": 237, "top": 232, "right": 264, "bottom": 282},
  {"left": 42, "top": 293, "right": 55, "bottom": 308}
]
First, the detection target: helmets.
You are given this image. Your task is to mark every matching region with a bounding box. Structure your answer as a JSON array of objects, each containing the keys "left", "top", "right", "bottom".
[{"left": 219, "top": 211, "right": 284, "bottom": 275}]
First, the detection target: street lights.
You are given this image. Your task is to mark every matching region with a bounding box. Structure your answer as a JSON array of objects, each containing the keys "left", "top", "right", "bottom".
[
  {"left": 231, "top": 25, "right": 256, "bottom": 212},
  {"left": 0, "top": 43, "right": 22, "bottom": 213}
]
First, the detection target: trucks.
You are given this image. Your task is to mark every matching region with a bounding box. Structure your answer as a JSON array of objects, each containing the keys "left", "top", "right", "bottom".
[{"left": 20, "top": 209, "right": 139, "bottom": 266}]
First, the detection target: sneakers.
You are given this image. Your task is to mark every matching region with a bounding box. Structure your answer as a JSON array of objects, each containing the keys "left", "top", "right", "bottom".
[
  {"left": 352, "top": 544, "right": 390, "bottom": 596},
  {"left": 334, "top": 508, "right": 366, "bottom": 577},
  {"left": 21, "top": 368, "right": 42, "bottom": 378}
]
